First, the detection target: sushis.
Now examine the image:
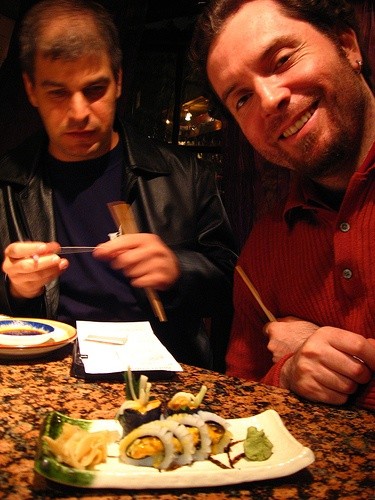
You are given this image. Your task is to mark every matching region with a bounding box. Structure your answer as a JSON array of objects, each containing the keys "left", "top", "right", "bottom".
[
  {"left": 118, "top": 410, "right": 233, "bottom": 470},
  {"left": 116, "top": 398, "right": 162, "bottom": 432},
  {"left": 165, "top": 391, "right": 200, "bottom": 417}
]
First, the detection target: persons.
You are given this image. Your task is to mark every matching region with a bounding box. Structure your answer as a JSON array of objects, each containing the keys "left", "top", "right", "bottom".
[
  {"left": 189, "top": 1, "right": 375, "bottom": 416},
  {"left": 1, "top": 0, "right": 240, "bottom": 374}
]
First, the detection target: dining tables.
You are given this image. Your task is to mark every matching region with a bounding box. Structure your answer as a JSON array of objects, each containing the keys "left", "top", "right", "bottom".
[{"left": 0, "top": 360, "right": 374, "bottom": 500}]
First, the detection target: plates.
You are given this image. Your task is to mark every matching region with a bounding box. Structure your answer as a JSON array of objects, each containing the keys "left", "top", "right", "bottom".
[
  {"left": 0, "top": 317, "right": 77, "bottom": 360},
  {"left": 33, "top": 410, "right": 316, "bottom": 489}
]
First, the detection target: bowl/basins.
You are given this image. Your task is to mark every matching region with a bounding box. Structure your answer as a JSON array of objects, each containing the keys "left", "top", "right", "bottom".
[{"left": 0, "top": 320, "right": 55, "bottom": 345}]
